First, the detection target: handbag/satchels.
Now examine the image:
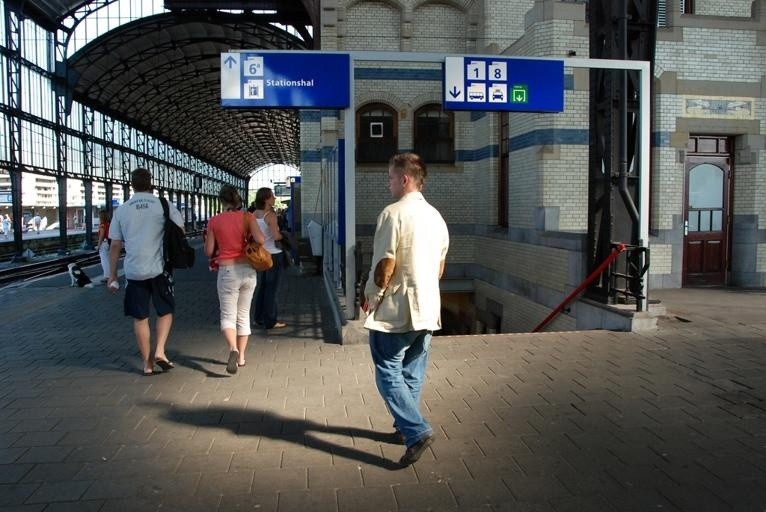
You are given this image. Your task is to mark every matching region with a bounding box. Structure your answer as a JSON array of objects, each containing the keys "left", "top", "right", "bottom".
[{"left": 163, "top": 219, "right": 195, "bottom": 270}]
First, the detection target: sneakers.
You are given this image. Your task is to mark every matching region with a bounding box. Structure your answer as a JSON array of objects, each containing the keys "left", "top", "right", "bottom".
[
  {"left": 253, "top": 321, "right": 287, "bottom": 327},
  {"left": 244, "top": 239, "right": 274, "bottom": 271},
  {"left": 101, "top": 278, "right": 110, "bottom": 282},
  {"left": 397, "top": 432, "right": 435, "bottom": 465}
]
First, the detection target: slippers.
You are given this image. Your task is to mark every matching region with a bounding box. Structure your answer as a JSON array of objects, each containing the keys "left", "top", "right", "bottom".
[
  {"left": 143, "top": 360, "right": 174, "bottom": 375},
  {"left": 227, "top": 349, "right": 245, "bottom": 374}
]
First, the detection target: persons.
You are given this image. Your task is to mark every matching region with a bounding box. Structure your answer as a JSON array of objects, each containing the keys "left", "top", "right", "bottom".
[
  {"left": 105, "top": 169, "right": 187, "bottom": 377},
  {"left": 359, "top": 154, "right": 453, "bottom": 466},
  {"left": 72, "top": 212, "right": 79, "bottom": 231},
  {"left": 34, "top": 213, "right": 42, "bottom": 234},
  {"left": 2, "top": 215, "right": 10, "bottom": 238},
  {"left": 236, "top": 197, "right": 289, "bottom": 236},
  {"left": 249, "top": 187, "right": 289, "bottom": 327},
  {"left": 201, "top": 184, "right": 266, "bottom": 375},
  {"left": 93, "top": 210, "right": 113, "bottom": 284}
]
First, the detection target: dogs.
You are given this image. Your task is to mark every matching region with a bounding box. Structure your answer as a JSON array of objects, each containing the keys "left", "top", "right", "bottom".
[{"left": 66, "top": 256, "right": 96, "bottom": 288}]
